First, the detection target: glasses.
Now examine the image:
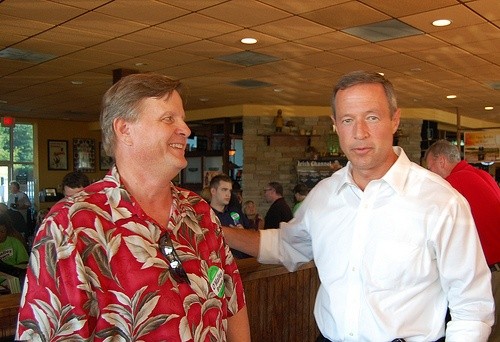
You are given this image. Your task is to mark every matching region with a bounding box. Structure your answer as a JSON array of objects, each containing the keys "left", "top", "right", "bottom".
[
  {"left": 158, "top": 230, "right": 191, "bottom": 285},
  {"left": 263, "top": 188, "right": 272, "bottom": 193}
]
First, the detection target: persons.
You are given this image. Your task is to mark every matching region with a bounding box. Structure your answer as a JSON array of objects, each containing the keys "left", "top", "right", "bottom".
[
  {"left": 242, "top": 200, "right": 264, "bottom": 231},
  {"left": 7, "top": 182, "right": 31, "bottom": 223},
  {"left": 62, "top": 171, "right": 89, "bottom": 198},
  {"left": 15, "top": 74, "right": 250, "bottom": 342},
  {"left": 222, "top": 70, "right": 496, "bottom": 342},
  {"left": 0, "top": 203, "right": 50, "bottom": 295},
  {"left": 263, "top": 182, "right": 294, "bottom": 229},
  {"left": 209, "top": 174, "right": 251, "bottom": 260},
  {"left": 426, "top": 139, "right": 500, "bottom": 342},
  {"left": 292, "top": 184, "right": 309, "bottom": 217}
]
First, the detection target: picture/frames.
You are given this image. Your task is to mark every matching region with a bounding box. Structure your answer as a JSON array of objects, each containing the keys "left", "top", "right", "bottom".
[
  {"left": 47, "top": 139, "right": 69, "bottom": 171},
  {"left": 99, "top": 142, "right": 112, "bottom": 171}
]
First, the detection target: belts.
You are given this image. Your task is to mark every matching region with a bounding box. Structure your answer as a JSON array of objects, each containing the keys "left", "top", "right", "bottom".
[{"left": 489, "top": 264, "right": 500, "bottom": 272}]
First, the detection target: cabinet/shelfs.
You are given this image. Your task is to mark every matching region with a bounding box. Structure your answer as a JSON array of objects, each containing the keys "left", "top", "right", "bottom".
[
  {"left": 420, "top": 119, "right": 440, "bottom": 165},
  {"left": 178, "top": 117, "right": 243, "bottom": 190}
]
